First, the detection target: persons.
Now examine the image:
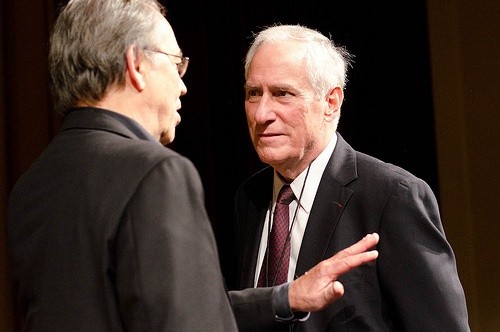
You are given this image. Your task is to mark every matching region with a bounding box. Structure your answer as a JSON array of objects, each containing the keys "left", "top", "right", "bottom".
[
  {"left": 232, "top": 23, "right": 471, "bottom": 331},
  {"left": 0, "top": 0, "right": 382, "bottom": 332}
]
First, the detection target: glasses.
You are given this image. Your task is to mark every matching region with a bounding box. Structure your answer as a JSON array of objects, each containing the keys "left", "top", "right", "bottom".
[{"left": 144, "top": 46, "right": 189, "bottom": 78}]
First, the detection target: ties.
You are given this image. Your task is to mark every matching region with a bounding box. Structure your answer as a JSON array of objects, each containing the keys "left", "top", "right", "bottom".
[{"left": 256, "top": 184, "right": 297, "bottom": 289}]
